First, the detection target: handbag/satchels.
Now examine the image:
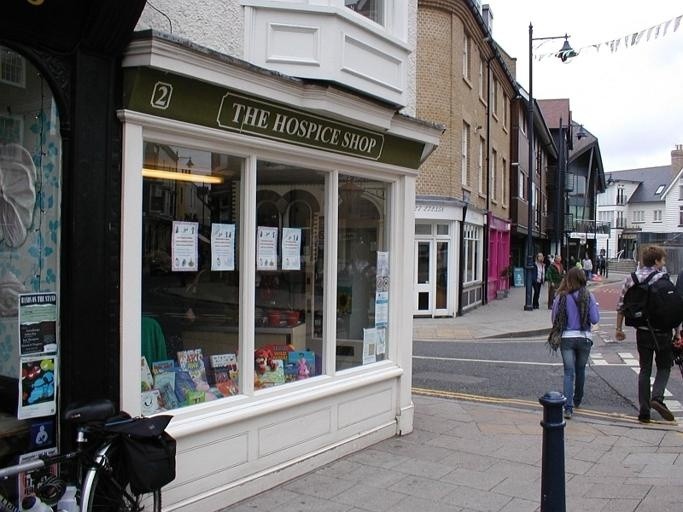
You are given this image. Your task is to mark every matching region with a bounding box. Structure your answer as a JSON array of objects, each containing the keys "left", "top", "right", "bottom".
[{"left": 94, "top": 412, "right": 175, "bottom": 493}]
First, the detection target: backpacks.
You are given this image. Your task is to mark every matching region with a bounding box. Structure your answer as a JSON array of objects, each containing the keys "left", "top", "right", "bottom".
[{"left": 624, "top": 270, "right": 683, "bottom": 330}]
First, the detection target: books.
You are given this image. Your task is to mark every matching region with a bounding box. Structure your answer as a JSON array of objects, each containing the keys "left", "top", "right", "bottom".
[{"left": 139, "top": 344, "right": 318, "bottom": 420}]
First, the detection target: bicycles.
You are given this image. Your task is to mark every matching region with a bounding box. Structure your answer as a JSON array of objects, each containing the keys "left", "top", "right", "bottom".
[{"left": 3, "top": 392, "right": 178, "bottom": 512}]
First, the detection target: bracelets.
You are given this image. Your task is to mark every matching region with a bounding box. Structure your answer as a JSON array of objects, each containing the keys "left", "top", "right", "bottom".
[{"left": 615, "top": 327, "right": 622, "bottom": 331}]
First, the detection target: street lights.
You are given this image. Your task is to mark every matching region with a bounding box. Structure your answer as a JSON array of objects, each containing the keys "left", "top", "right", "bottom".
[
  {"left": 554, "top": 112, "right": 587, "bottom": 262},
  {"left": 523, "top": 20, "right": 574, "bottom": 312}
]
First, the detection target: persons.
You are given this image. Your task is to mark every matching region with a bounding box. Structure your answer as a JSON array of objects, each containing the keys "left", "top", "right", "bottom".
[
  {"left": 551, "top": 265, "right": 600, "bottom": 419},
  {"left": 531, "top": 249, "right": 606, "bottom": 311},
  {"left": 613, "top": 246, "right": 682, "bottom": 424}
]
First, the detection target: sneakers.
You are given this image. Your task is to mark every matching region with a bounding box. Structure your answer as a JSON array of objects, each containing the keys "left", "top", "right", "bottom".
[
  {"left": 638, "top": 415, "right": 651, "bottom": 423},
  {"left": 650, "top": 398, "right": 674, "bottom": 421},
  {"left": 563, "top": 409, "right": 573, "bottom": 418}
]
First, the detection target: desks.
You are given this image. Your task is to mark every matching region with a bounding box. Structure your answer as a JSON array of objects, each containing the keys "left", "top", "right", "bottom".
[{"left": 182, "top": 322, "right": 306, "bottom": 352}]
